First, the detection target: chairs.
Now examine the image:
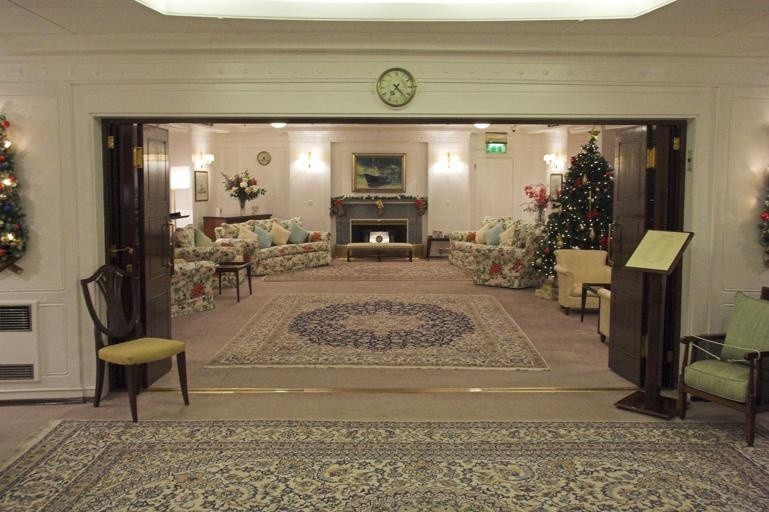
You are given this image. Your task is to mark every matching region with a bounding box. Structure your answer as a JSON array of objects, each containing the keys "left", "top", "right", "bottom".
[
  {"left": 597, "top": 287, "right": 612, "bottom": 341},
  {"left": 170, "top": 258, "right": 220, "bottom": 319},
  {"left": 676, "top": 286, "right": 769, "bottom": 445},
  {"left": 172, "top": 224, "right": 246, "bottom": 288},
  {"left": 80, "top": 265, "right": 190, "bottom": 423},
  {"left": 554, "top": 248, "right": 613, "bottom": 315}
]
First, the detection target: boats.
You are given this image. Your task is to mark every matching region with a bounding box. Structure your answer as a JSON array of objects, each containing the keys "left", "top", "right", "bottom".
[{"left": 364, "top": 166, "right": 393, "bottom": 185}]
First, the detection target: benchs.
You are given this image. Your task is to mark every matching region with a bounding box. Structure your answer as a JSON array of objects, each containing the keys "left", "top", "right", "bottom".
[{"left": 346, "top": 242, "right": 413, "bottom": 262}]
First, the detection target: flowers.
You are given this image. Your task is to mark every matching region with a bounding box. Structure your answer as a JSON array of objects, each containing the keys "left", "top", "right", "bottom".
[
  {"left": 518, "top": 183, "right": 551, "bottom": 221},
  {"left": 220, "top": 168, "right": 266, "bottom": 207}
]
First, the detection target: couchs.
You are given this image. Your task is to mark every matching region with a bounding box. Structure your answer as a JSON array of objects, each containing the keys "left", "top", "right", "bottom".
[
  {"left": 449, "top": 226, "right": 548, "bottom": 289},
  {"left": 214, "top": 227, "right": 333, "bottom": 277}
]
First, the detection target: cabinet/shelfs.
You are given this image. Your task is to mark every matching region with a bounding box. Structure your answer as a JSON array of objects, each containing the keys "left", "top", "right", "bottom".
[{"left": 203, "top": 214, "right": 272, "bottom": 241}]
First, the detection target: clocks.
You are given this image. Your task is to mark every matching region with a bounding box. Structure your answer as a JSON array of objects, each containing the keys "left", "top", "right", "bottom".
[
  {"left": 257, "top": 151, "right": 272, "bottom": 166},
  {"left": 376, "top": 67, "right": 417, "bottom": 107}
]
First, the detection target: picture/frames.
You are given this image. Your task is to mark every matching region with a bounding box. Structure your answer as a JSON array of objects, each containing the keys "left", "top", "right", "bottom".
[
  {"left": 351, "top": 152, "right": 407, "bottom": 194},
  {"left": 194, "top": 170, "right": 209, "bottom": 201},
  {"left": 550, "top": 173, "right": 563, "bottom": 204}
]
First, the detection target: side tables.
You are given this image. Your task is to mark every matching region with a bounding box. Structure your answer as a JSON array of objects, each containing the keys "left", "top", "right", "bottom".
[{"left": 426, "top": 234, "right": 451, "bottom": 261}]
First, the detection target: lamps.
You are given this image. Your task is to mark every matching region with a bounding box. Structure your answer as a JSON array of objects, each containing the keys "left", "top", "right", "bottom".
[{"left": 170, "top": 165, "right": 192, "bottom": 229}]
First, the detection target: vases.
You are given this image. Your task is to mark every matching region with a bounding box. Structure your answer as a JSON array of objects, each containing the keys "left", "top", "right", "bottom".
[{"left": 239, "top": 199, "right": 246, "bottom": 216}]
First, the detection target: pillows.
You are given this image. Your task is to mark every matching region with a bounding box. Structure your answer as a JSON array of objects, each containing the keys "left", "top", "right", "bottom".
[
  {"left": 174, "top": 226, "right": 195, "bottom": 248},
  {"left": 221, "top": 216, "right": 310, "bottom": 249},
  {"left": 720, "top": 290, "right": 769, "bottom": 364},
  {"left": 475, "top": 215, "right": 543, "bottom": 249}
]
